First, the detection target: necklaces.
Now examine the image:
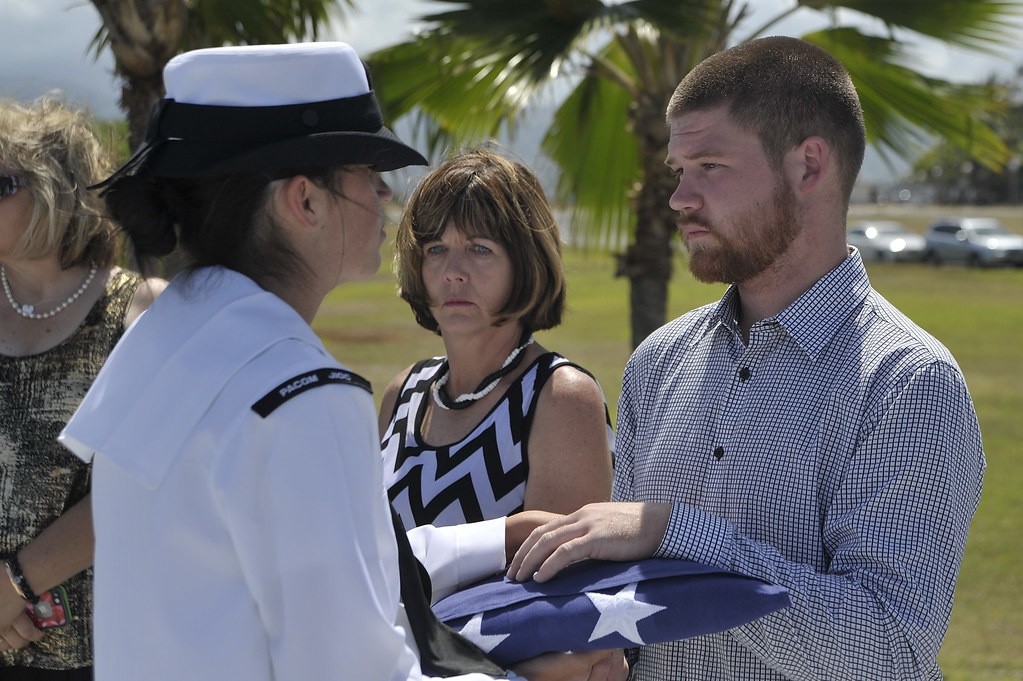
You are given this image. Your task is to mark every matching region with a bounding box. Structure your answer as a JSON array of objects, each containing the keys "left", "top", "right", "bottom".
[
  {"left": 0, "top": 258, "right": 103, "bottom": 319},
  {"left": 431, "top": 334, "right": 535, "bottom": 411}
]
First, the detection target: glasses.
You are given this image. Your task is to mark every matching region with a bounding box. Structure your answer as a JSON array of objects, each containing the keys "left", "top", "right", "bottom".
[{"left": 0, "top": 174, "right": 28, "bottom": 199}]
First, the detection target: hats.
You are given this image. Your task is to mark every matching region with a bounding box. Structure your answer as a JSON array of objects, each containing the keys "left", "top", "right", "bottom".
[{"left": 85, "top": 42, "right": 428, "bottom": 197}]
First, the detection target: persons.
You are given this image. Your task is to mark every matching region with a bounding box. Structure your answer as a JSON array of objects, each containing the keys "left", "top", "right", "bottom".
[
  {"left": 499, "top": 31, "right": 985, "bottom": 681},
  {"left": 52, "top": 40, "right": 640, "bottom": 681},
  {"left": 361, "top": 143, "right": 619, "bottom": 580},
  {"left": 0, "top": 85, "right": 182, "bottom": 681}
]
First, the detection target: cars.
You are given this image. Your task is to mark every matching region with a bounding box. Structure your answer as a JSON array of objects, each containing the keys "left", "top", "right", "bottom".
[
  {"left": 923, "top": 217, "right": 1023, "bottom": 270},
  {"left": 847, "top": 219, "right": 931, "bottom": 264}
]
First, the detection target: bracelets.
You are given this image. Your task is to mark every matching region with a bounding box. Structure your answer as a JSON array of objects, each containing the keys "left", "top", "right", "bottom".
[{"left": 3, "top": 548, "right": 42, "bottom": 605}]
